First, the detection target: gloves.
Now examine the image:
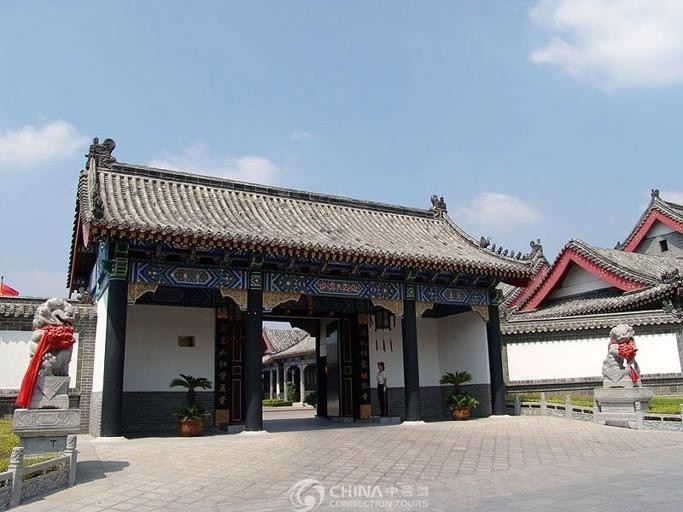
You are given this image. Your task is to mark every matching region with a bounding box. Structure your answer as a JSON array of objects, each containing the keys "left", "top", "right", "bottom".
[{"left": 383, "top": 387, "right": 386, "bottom": 392}]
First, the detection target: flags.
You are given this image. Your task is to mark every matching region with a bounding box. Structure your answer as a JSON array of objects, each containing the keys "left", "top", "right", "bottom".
[{"left": 0, "top": 284, "right": 19, "bottom": 296}]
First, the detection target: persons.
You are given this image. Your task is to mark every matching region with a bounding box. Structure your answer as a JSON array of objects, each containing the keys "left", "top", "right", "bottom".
[{"left": 377, "top": 362, "right": 388, "bottom": 416}]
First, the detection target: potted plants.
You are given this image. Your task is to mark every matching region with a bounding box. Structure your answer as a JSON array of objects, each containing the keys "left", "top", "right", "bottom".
[
  {"left": 440, "top": 370, "right": 479, "bottom": 420},
  {"left": 168, "top": 372, "right": 212, "bottom": 437}
]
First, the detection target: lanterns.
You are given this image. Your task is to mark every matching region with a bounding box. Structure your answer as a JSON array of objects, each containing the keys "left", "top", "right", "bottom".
[{"left": 375, "top": 310, "right": 392, "bottom": 331}]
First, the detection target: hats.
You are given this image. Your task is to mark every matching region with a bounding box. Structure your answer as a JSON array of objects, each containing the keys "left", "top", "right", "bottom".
[{"left": 377, "top": 362, "right": 384, "bottom": 366}]
